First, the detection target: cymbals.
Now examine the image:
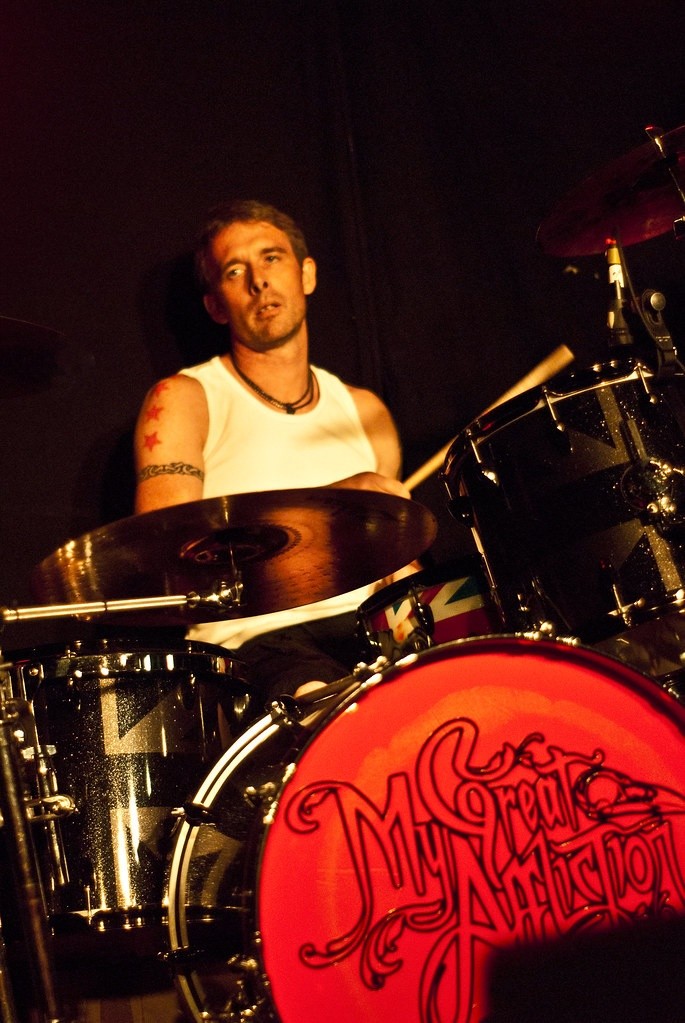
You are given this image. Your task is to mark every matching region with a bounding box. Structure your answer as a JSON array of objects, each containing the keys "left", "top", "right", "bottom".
[
  {"left": 535, "top": 124, "right": 684, "bottom": 256},
  {"left": 30, "top": 487, "right": 436, "bottom": 627}
]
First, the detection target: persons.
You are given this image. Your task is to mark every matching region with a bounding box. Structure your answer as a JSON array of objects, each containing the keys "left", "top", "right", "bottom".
[{"left": 131, "top": 195, "right": 414, "bottom": 676}]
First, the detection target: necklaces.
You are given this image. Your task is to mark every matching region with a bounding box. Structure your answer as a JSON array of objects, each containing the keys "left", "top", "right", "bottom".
[{"left": 229, "top": 351, "right": 317, "bottom": 415}]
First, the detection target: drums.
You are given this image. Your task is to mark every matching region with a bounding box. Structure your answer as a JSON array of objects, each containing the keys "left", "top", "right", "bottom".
[{"left": 16, "top": 348, "right": 685, "bottom": 1023}]
